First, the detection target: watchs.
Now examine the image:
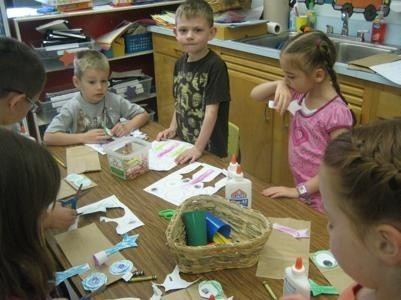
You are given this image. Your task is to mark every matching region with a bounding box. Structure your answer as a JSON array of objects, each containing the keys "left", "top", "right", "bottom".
[{"left": 296, "top": 182, "right": 310, "bottom": 200}]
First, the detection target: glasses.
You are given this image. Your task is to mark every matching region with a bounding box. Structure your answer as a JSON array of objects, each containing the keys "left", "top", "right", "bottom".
[{"left": 27, "top": 97, "right": 39, "bottom": 113}]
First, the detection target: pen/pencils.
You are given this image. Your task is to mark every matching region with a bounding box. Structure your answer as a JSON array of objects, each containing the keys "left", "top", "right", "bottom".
[
  {"left": 48, "top": 151, "right": 68, "bottom": 171},
  {"left": 129, "top": 274, "right": 157, "bottom": 282},
  {"left": 212, "top": 232, "right": 231, "bottom": 244},
  {"left": 95, "top": 113, "right": 116, "bottom": 141},
  {"left": 263, "top": 281, "right": 279, "bottom": 299}
]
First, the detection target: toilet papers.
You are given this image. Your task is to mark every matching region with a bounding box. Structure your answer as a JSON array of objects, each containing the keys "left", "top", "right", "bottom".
[{"left": 267, "top": 21, "right": 281, "bottom": 35}]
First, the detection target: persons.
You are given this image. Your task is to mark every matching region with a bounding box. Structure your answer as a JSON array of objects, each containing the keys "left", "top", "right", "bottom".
[
  {"left": 250, "top": 29, "right": 357, "bottom": 218},
  {"left": 1, "top": 34, "right": 79, "bottom": 237},
  {"left": 0, "top": 126, "right": 62, "bottom": 300},
  {"left": 154, "top": 0, "right": 232, "bottom": 166},
  {"left": 42, "top": 48, "right": 152, "bottom": 147},
  {"left": 280, "top": 117, "right": 400, "bottom": 300}
]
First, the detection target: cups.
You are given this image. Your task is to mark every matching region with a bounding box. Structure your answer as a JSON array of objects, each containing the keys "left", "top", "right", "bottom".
[
  {"left": 204, "top": 211, "right": 231, "bottom": 244},
  {"left": 296, "top": 14, "right": 308, "bottom": 32},
  {"left": 182, "top": 209, "right": 209, "bottom": 246}
]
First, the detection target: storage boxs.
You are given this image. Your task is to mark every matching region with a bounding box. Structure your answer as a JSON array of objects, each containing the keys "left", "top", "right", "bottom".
[
  {"left": 30, "top": 33, "right": 95, "bottom": 69},
  {"left": 37, "top": 90, "right": 81, "bottom": 123},
  {"left": 110, "top": 74, "right": 152, "bottom": 103},
  {"left": 143, "top": 107, "right": 156, "bottom": 123}
]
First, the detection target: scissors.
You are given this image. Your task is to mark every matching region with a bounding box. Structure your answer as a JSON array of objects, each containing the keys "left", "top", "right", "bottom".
[
  {"left": 159, "top": 209, "right": 176, "bottom": 220},
  {"left": 61, "top": 184, "right": 92, "bottom": 209}
]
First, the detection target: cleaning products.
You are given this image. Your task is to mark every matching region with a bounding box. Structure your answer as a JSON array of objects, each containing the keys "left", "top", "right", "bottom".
[{"left": 369, "top": 5, "right": 387, "bottom": 42}]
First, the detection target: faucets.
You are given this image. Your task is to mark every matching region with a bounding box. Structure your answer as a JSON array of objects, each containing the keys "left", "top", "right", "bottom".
[{"left": 340, "top": 6, "right": 349, "bottom": 35}]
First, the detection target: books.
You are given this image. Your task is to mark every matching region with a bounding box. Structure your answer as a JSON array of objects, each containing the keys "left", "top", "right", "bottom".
[
  {"left": 44, "top": 68, "right": 148, "bottom": 120},
  {"left": 36, "top": 19, "right": 90, "bottom": 59}
]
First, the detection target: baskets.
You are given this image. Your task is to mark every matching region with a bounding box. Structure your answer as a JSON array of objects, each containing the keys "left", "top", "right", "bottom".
[
  {"left": 124, "top": 32, "right": 152, "bottom": 53},
  {"left": 165, "top": 194, "right": 273, "bottom": 274}
]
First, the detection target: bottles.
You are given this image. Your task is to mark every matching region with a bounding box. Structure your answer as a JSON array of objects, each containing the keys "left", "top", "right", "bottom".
[
  {"left": 282, "top": 256, "right": 313, "bottom": 300},
  {"left": 223, "top": 153, "right": 254, "bottom": 210}
]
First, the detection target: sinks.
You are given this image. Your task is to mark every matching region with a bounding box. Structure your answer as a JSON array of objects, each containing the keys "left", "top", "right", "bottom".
[{"left": 234, "top": 30, "right": 400, "bottom": 66}]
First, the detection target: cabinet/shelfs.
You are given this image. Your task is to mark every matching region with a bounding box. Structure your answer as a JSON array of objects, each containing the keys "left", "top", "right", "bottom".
[
  {"left": 148, "top": 32, "right": 204, "bottom": 144},
  {"left": 364, "top": 81, "right": 401, "bottom": 120},
  {"left": 205, "top": 44, "right": 364, "bottom": 203},
  {"left": 10, "top": 0, "right": 202, "bottom": 144}
]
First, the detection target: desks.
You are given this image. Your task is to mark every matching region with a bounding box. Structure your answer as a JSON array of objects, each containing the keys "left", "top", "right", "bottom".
[{"left": 36, "top": 121, "right": 357, "bottom": 300}]
